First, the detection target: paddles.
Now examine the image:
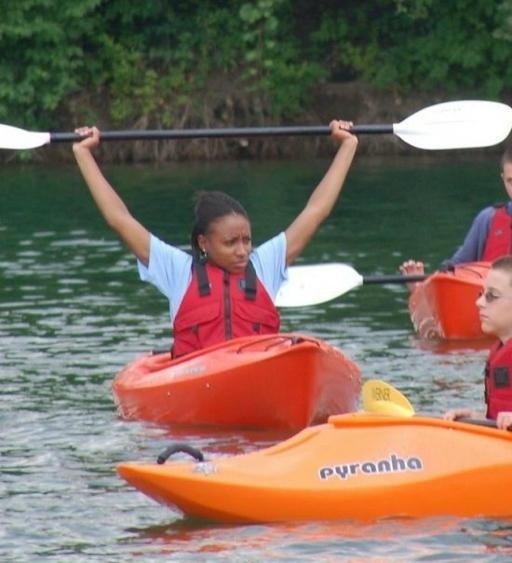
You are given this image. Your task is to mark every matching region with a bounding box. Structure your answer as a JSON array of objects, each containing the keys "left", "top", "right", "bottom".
[
  {"left": 361, "top": 378, "right": 496, "bottom": 427},
  {"left": 275, "top": 262, "right": 428, "bottom": 308},
  {"left": 0, "top": 97, "right": 512, "bottom": 151}
]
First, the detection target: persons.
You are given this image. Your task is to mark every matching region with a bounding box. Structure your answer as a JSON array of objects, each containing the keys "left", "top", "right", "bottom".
[
  {"left": 399, "top": 142, "right": 512, "bottom": 293},
  {"left": 442, "top": 253, "right": 512, "bottom": 431},
  {"left": 72, "top": 119, "right": 358, "bottom": 359}
]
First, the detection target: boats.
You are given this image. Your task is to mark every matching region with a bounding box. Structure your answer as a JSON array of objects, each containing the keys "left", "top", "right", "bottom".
[
  {"left": 406, "top": 259, "right": 508, "bottom": 352},
  {"left": 109, "top": 330, "right": 367, "bottom": 440},
  {"left": 114, "top": 409, "right": 512, "bottom": 527}
]
